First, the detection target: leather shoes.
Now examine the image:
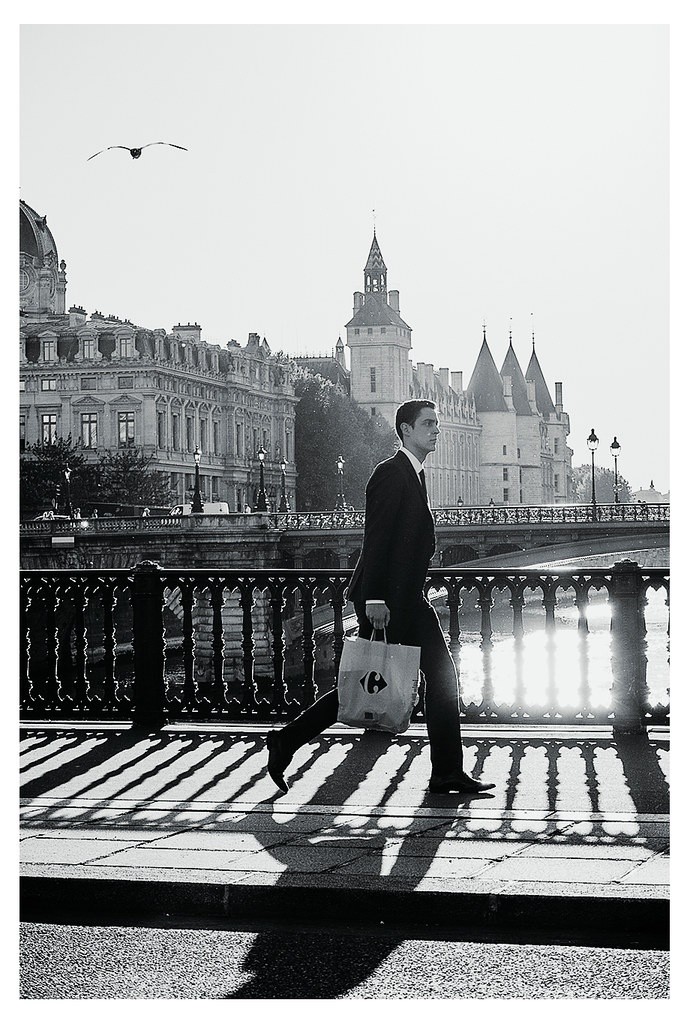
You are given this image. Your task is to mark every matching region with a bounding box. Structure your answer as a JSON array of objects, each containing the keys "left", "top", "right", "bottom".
[
  {"left": 265, "top": 729, "right": 295, "bottom": 795},
  {"left": 428, "top": 768, "right": 497, "bottom": 792}
]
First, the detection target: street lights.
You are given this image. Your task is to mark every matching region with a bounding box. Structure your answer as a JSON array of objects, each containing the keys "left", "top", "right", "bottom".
[
  {"left": 278, "top": 455, "right": 290, "bottom": 512},
  {"left": 586, "top": 429, "right": 599, "bottom": 504},
  {"left": 336, "top": 455, "right": 347, "bottom": 510},
  {"left": 610, "top": 437, "right": 621, "bottom": 503},
  {"left": 192, "top": 445, "right": 205, "bottom": 513},
  {"left": 62, "top": 463, "right": 73, "bottom": 517},
  {"left": 257, "top": 445, "right": 268, "bottom": 511}
]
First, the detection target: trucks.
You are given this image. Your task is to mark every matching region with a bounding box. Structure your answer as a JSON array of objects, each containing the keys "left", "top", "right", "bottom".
[{"left": 161, "top": 502, "right": 230, "bottom": 525}]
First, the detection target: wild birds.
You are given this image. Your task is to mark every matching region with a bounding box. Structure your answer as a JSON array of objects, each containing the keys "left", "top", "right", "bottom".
[{"left": 87, "top": 141, "right": 187, "bottom": 161}]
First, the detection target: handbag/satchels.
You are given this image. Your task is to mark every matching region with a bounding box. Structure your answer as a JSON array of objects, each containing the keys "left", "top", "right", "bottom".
[{"left": 336, "top": 621, "right": 421, "bottom": 734}]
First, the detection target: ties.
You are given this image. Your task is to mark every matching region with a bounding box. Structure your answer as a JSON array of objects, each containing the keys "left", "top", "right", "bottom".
[{"left": 418, "top": 468, "right": 428, "bottom": 503}]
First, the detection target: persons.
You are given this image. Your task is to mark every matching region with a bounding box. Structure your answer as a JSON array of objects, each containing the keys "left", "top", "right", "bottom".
[
  {"left": 144, "top": 507, "right": 150, "bottom": 516},
  {"left": 267, "top": 399, "right": 496, "bottom": 795},
  {"left": 245, "top": 503, "right": 251, "bottom": 513},
  {"left": 92, "top": 509, "right": 97, "bottom": 517},
  {"left": 73, "top": 508, "right": 81, "bottom": 518}
]
